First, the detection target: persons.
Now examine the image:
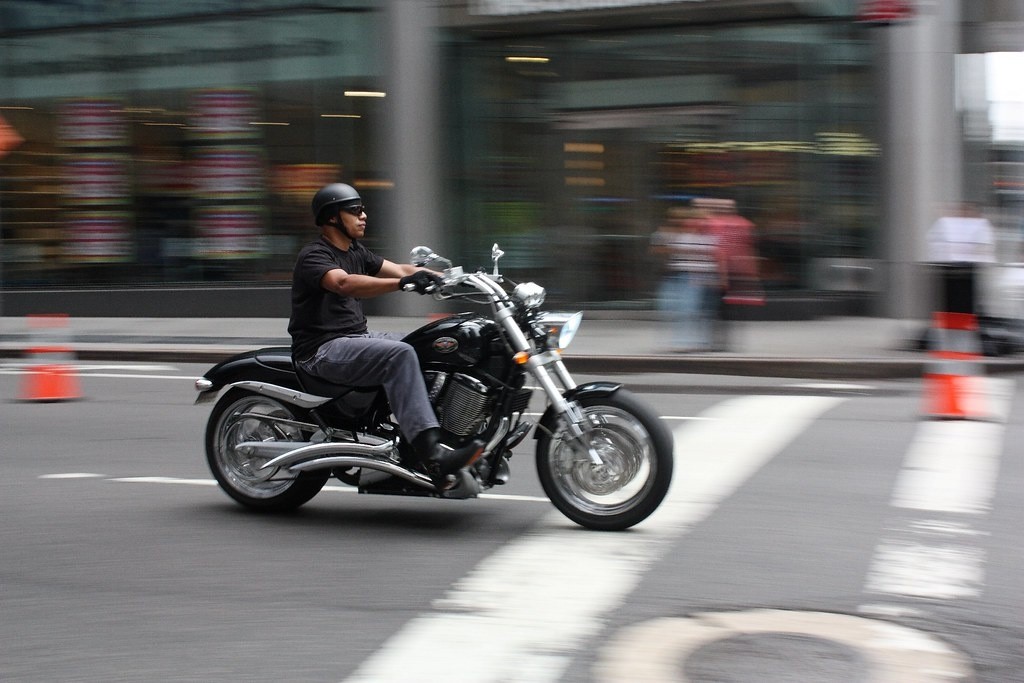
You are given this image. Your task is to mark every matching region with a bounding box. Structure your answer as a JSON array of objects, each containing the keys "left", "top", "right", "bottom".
[{"left": 289, "top": 184, "right": 485, "bottom": 493}]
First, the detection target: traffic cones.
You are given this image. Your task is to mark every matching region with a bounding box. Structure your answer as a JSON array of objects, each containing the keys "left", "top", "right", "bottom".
[
  {"left": 18, "top": 313, "right": 84, "bottom": 405},
  {"left": 917, "top": 310, "right": 995, "bottom": 423}
]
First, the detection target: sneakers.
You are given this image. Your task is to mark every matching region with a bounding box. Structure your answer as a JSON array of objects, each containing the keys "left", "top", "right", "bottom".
[{"left": 420, "top": 440, "right": 487, "bottom": 492}]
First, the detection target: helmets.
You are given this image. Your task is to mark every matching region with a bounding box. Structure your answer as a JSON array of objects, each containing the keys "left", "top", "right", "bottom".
[{"left": 313, "top": 183, "right": 364, "bottom": 227}]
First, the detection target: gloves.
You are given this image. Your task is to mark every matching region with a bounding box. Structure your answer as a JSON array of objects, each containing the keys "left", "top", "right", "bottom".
[{"left": 399, "top": 270, "right": 441, "bottom": 297}]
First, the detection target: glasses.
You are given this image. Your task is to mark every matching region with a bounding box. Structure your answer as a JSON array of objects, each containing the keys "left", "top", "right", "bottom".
[{"left": 343, "top": 204, "right": 367, "bottom": 214}]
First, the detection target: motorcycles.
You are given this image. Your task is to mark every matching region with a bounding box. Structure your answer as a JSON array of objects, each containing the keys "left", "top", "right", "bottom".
[{"left": 194, "top": 243, "right": 675, "bottom": 531}]
